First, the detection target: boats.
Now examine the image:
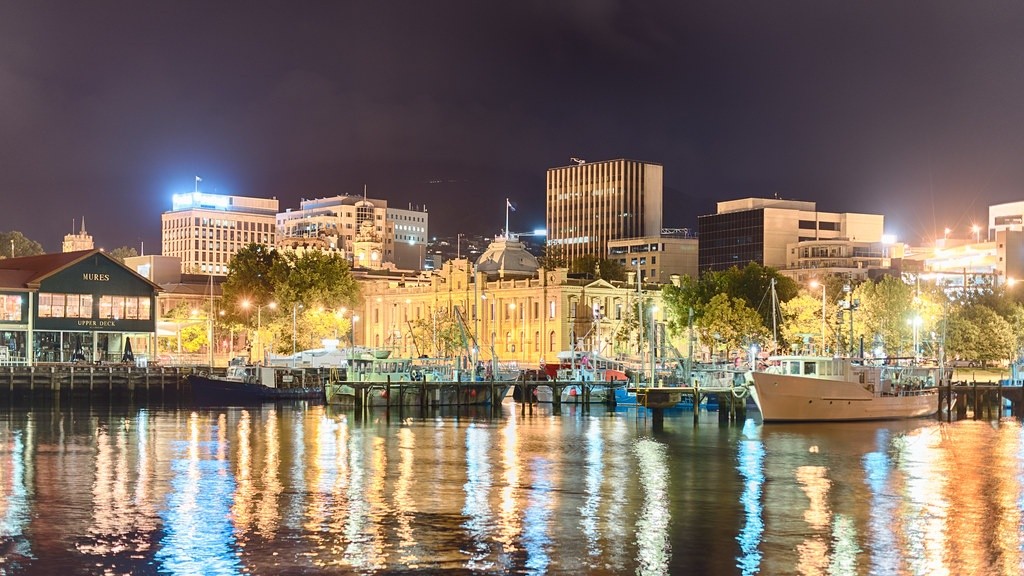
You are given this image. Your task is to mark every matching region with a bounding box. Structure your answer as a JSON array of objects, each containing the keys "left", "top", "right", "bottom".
[
  {"left": 325, "top": 305, "right": 518, "bottom": 404},
  {"left": 533, "top": 324, "right": 618, "bottom": 402},
  {"left": 187, "top": 366, "right": 325, "bottom": 402},
  {"left": 743, "top": 356, "right": 939, "bottom": 421}
]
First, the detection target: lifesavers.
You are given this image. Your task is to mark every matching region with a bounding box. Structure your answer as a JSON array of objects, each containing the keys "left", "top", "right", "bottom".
[{"left": 867, "top": 384, "right": 874, "bottom": 393}]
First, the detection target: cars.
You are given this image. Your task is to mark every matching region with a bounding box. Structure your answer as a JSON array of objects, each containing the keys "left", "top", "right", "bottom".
[{"left": 947, "top": 358, "right": 980, "bottom": 368}]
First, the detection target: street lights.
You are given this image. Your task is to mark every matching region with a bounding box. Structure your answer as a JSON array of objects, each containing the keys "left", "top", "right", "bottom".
[
  {"left": 651, "top": 306, "right": 659, "bottom": 387},
  {"left": 810, "top": 282, "right": 826, "bottom": 356},
  {"left": 243, "top": 301, "right": 276, "bottom": 366}
]
